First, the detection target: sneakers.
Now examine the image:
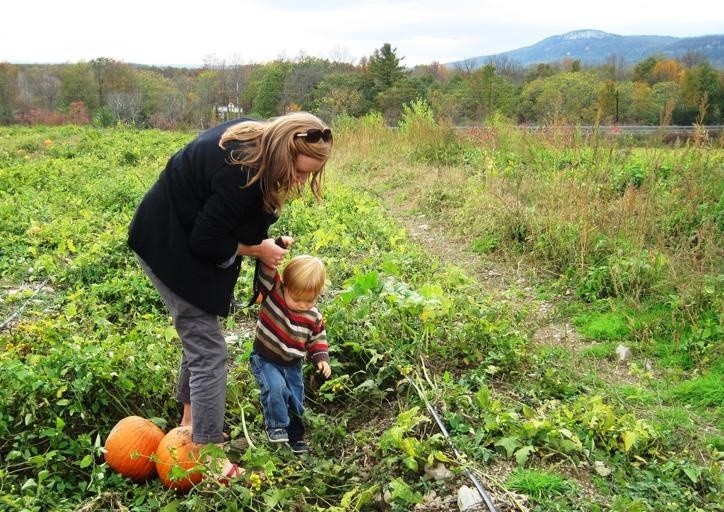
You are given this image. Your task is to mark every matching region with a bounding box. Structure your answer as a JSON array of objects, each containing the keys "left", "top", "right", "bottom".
[
  {"left": 265, "top": 425, "right": 289, "bottom": 442},
  {"left": 285, "top": 440, "right": 309, "bottom": 453}
]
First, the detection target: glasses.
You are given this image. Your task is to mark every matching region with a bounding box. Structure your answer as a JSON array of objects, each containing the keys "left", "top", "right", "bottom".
[{"left": 296, "top": 128, "right": 331, "bottom": 144}]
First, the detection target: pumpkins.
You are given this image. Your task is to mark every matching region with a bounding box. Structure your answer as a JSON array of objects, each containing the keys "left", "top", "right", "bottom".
[
  {"left": 103, "top": 416, "right": 166, "bottom": 481},
  {"left": 156, "top": 426, "right": 209, "bottom": 490}
]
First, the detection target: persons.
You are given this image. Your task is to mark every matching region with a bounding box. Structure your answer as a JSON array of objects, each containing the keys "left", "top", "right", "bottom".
[
  {"left": 248, "top": 235, "right": 333, "bottom": 455},
  {"left": 127, "top": 111, "right": 333, "bottom": 479}
]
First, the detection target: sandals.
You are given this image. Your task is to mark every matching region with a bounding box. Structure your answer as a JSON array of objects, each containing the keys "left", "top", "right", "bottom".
[{"left": 205, "top": 467, "right": 263, "bottom": 489}]
